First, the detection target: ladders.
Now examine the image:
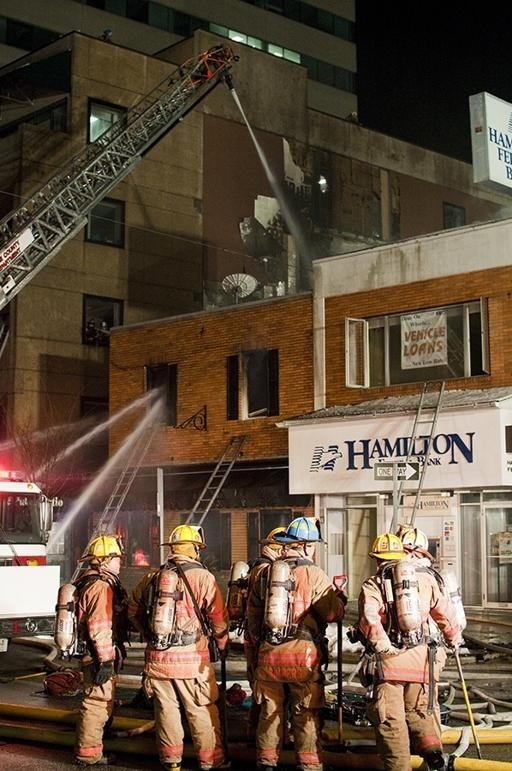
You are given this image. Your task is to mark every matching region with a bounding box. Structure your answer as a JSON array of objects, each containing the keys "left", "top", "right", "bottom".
[
  {"left": 184, "top": 436, "right": 248, "bottom": 526},
  {"left": 389, "top": 382, "right": 446, "bottom": 532},
  {"left": 70, "top": 419, "right": 156, "bottom": 582}
]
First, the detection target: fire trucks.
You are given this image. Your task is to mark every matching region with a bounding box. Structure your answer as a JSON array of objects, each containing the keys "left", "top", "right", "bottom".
[{"left": 0, "top": 43, "right": 240, "bottom": 654}]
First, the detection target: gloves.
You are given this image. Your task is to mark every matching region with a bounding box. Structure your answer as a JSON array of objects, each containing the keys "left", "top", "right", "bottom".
[{"left": 92, "top": 661, "right": 113, "bottom": 686}]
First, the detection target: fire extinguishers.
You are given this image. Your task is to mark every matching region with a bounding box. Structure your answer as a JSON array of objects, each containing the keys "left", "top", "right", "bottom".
[
  {"left": 438, "top": 571, "right": 467, "bottom": 631},
  {"left": 225, "top": 561, "right": 249, "bottom": 630},
  {"left": 152, "top": 571, "right": 178, "bottom": 646},
  {"left": 54, "top": 585, "right": 76, "bottom": 659},
  {"left": 382, "top": 561, "right": 422, "bottom": 647},
  {"left": 264, "top": 561, "right": 290, "bottom": 645}
]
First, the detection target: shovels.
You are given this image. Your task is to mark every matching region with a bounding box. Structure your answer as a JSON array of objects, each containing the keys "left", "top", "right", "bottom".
[{"left": 327, "top": 576, "right": 349, "bottom": 751}]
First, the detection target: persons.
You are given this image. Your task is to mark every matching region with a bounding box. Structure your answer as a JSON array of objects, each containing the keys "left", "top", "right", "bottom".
[
  {"left": 87, "top": 318, "right": 109, "bottom": 344},
  {"left": 239, "top": 528, "right": 292, "bottom": 753},
  {"left": 345, "top": 524, "right": 462, "bottom": 771},
  {"left": 76, "top": 536, "right": 128, "bottom": 770},
  {"left": 346, "top": 110, "right": 364, "bottom": 125},
  {"left": 98, "top": 29, "right": 113, "bottom": 42},
  {"left": 248, "top": 516, "right": 349, "bottom": 771},
  {"left": 131, "top": 525, "right": 234, "bottom": 770}
]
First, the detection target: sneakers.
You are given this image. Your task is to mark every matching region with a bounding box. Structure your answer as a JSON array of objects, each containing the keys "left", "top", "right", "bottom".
[
  {"left": 425, "top": 749, "right": 445, "bottom": 770},
  {"left": 75, "top": 753, "right": 109, "bottom": 766}
]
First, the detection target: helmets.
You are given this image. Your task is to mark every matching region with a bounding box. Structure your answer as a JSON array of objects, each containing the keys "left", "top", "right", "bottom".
[
  {"left": 88, "top": 534, "right": 124, "bottom": 564},
  {"left": 162, "top": 525, "right": 207, "bottom": 550},
  {"left": 367, "top": 532, "right": 406, "bottom": 561},
  {"left": 266, "top": 526, "right": 287, "bottom": 545},
  {"left": 285, "top": 516, "right": 322, "bottom": 542},
  {"left": 398, "top": 527, "right": 429, "bottom": 551}
]
